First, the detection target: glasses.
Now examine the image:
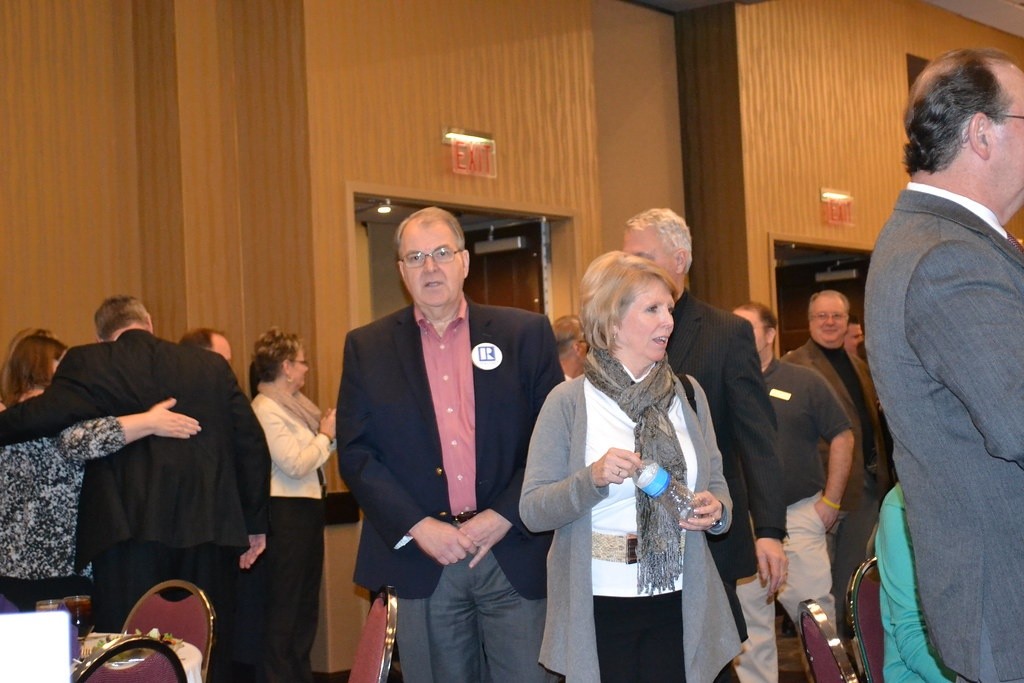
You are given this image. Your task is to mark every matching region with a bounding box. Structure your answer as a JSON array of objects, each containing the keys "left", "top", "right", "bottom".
[
  {"left": 400, "top": 246, "right": 462, "bottom": 268},
  {"left": 290, "top": 360, "right": 309, "bottom": 367},
  {"left": 810, "top": 312, "right": 845, "bottom": 321}
]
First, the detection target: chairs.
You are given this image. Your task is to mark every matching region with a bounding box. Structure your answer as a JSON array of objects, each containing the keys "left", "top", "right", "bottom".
[
  {"left": 71, "top": 580, "right": 399, "bottom": 683},
  {"left": 799, "top": 556, "right": 885, "bottom": 683}
]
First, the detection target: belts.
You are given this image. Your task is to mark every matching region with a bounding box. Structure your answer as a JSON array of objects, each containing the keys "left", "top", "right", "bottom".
[{"left": 450, "top": 509, "right": 477, "bottom": 525}]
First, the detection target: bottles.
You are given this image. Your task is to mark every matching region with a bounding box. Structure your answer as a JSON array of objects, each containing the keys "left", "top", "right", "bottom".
[{"left": 630, "top": 458, "right": 699, "bottom": 525}]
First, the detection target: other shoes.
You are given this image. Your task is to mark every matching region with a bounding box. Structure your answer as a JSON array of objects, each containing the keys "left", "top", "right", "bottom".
[{"left": 782, "top": 612, "right": 797, "bottom": 637}]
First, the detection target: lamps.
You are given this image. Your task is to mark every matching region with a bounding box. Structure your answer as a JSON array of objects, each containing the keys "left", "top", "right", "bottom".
[{"left": 377, "top": 202, "right": 391, "bottom": 213}]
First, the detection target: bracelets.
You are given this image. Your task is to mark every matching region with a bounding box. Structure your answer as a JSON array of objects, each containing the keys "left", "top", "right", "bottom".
[
  {"left": 822, "top": 493, "right": 842, "bottom": 511},
  {"left": 319, "top": 431, "right": 333, "bottom": 445}
]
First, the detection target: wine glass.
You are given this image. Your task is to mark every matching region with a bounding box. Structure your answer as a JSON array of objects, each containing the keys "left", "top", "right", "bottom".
[{"left": 64, "top": 594, "right": 96, "bottom": 666}]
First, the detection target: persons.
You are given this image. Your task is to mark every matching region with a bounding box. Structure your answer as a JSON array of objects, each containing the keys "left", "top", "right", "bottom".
[
  {"left": 518, "top": 249, "right": 744, "bottom": 683},
  {"left": 336, "top": 203, "right": 565, "bottom": 683},
  {"left": 779, "top": 47, "right": 1024, "bottom": 683},
  {"left": 0, "top": 295, "right": 338, "bottom": 682},
  {"left": 730, "top": 301, "right": 854, "bottom": 683},
  {"left": 619, "top": 208, "right": 789, "bottom": 683}
]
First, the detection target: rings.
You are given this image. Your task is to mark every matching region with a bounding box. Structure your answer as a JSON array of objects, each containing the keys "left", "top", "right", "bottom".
[{"left": 711, "top": 516, "right": 717, "bottom": 528}]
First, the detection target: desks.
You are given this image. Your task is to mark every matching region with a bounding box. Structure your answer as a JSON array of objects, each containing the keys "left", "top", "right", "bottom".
[{"left": 80, "top": 630, "right": 203, "bottom": 683}]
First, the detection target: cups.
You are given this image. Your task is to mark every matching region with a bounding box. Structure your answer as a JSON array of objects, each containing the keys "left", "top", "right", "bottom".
[{"left": 35, "top": 599, "right": 64, "bottom": 613}]
